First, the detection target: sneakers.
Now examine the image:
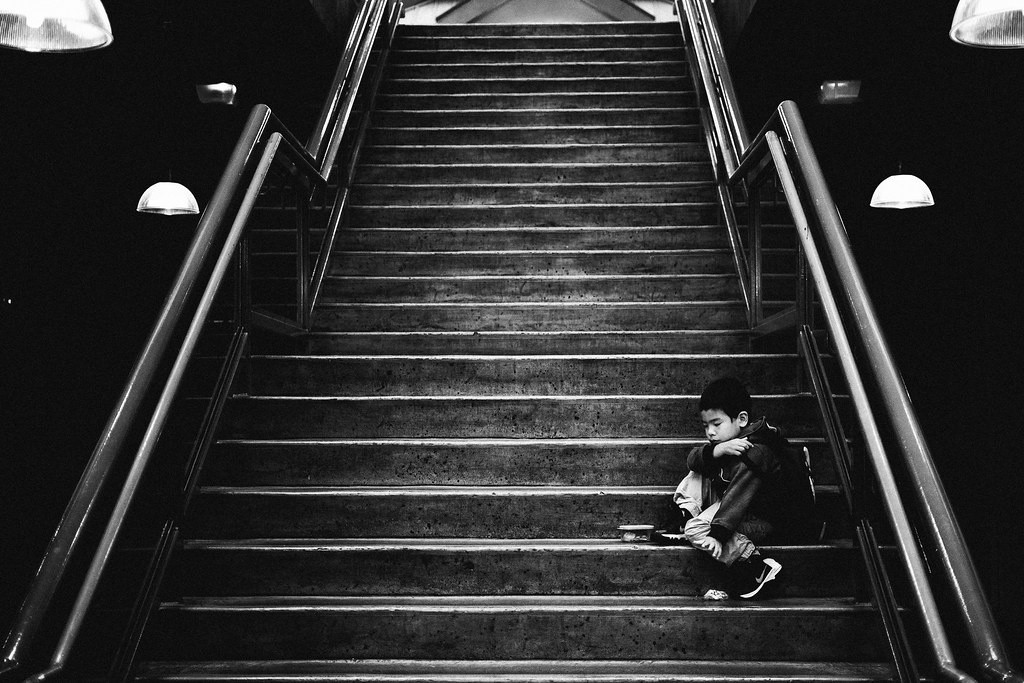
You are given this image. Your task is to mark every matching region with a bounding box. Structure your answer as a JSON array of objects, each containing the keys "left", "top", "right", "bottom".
[
  {"left": 661, "top": 508, "right": 693, "bottom": 538},
  {"left": 740, "top": 558, "right": 782, "bottom": 599}
]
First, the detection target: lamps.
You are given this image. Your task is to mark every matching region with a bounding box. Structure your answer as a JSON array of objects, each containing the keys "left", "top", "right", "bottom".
[
  {"left": 136, "top": 181, "right": 201, "bottom": 216},
  {"left": 0, "top": 0, "right": 114, "bottom": 54},
  {"left": 948, "top": 0, "right": 1024, "bottom": 50},
  {"left": 869, "top": 174, "right": 935, "bottom": 210}
]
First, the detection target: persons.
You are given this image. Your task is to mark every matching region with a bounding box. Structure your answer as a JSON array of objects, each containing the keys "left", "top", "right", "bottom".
[{"left": 648, "top": 377, "right": 828, "bottom": 601}]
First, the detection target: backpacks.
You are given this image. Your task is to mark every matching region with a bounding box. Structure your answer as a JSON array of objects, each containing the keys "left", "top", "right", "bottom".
[{"left": 739, "top": 435, "right": 827, "bottom": 544}]
